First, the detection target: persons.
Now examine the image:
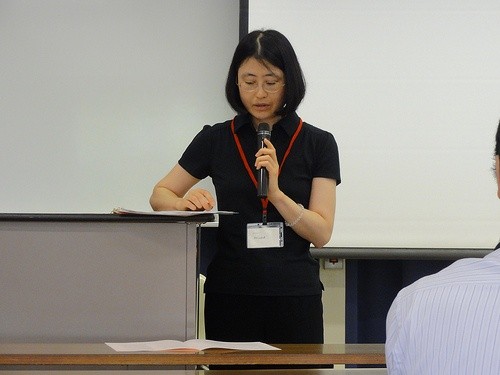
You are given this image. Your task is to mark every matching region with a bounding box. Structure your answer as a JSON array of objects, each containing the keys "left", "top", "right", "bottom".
[
  {"left": 384, "top": 123, "right": 499, "bottom": 375},
  {"left": 148, "top": 29, "right": 341, "bottom": 371}
]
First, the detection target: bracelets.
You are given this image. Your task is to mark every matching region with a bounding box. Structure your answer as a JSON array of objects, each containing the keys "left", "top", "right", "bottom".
[{"left": 285, "top": 200, "right": 305, "bottom": 233}]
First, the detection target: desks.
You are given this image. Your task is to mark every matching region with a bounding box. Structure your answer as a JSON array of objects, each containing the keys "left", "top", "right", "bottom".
[{"left": 0, "top": 344, "right": 386, "bottom": 365}]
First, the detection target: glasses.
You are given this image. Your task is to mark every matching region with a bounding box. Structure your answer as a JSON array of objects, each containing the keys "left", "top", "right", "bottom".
[{"left": 236, "top": 76, "right": 286, "bottom": 93}]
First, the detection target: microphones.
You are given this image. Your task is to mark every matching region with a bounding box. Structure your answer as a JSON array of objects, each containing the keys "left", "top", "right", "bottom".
[{"left": 257, "top": 123, "right": 271, "bottom": 198}]
